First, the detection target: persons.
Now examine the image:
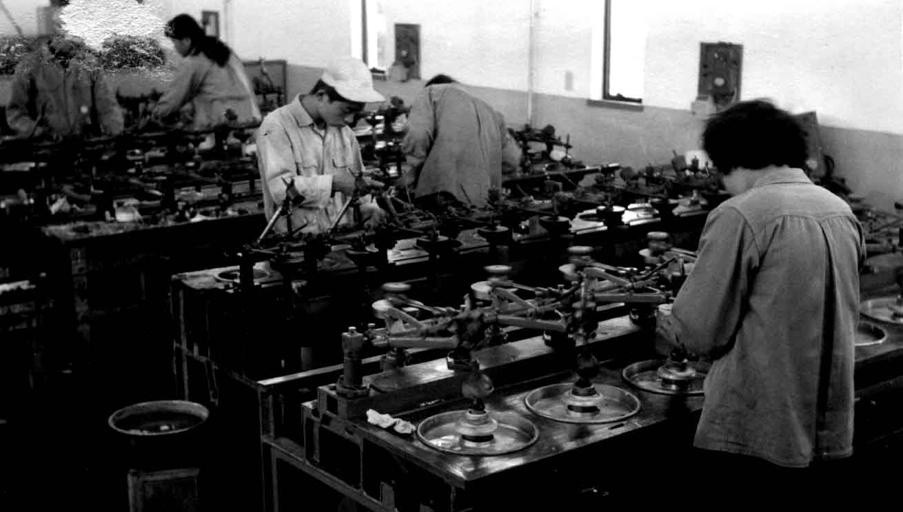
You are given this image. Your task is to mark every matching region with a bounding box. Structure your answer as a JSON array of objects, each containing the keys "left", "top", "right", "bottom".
[
  {"left": 402, "top": 73, "right": 521, "bottom": 209},
  {"left": 7, "top": 1, "right": 124, "bottom": 140},
  {"left": 258, "top": 55, "right": 384, "bottom": 237},
  {"left": 148, "top": 14, "right": 263, "bottom": 128},
  {"left": 655, "top": 100, "right": 867, "bottom": 512}
]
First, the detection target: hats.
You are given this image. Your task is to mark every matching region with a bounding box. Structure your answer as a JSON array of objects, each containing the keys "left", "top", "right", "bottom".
[{"left": 318, "top": 54, "right": 387, "bottom": 104}]
